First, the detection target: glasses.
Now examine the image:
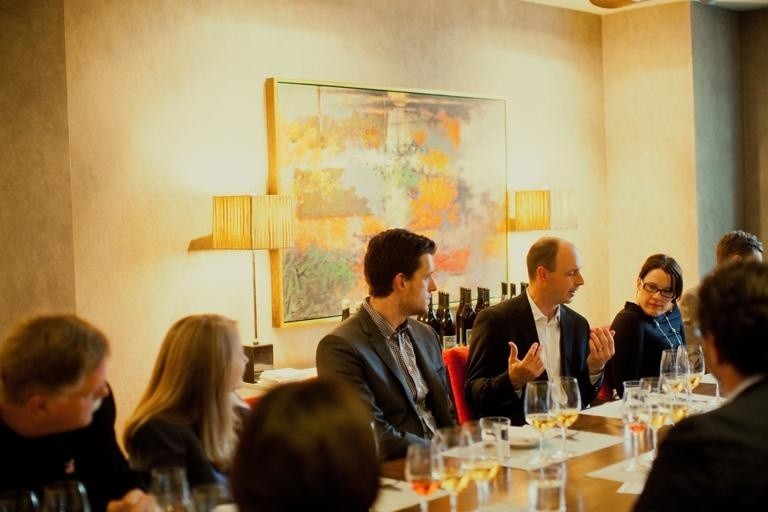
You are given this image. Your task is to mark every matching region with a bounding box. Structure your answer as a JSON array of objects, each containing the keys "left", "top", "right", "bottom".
[{"left": 639, "top": 278, "right": 677, "bottom": 299}]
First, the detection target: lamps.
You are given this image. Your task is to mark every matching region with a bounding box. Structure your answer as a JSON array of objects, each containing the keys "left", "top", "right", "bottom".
[
  {"left": 514, "top": 190, "right": 579, "bottom": 238},
  {"left": 209, "top": 193, "right": 297, "bottom": 377}
]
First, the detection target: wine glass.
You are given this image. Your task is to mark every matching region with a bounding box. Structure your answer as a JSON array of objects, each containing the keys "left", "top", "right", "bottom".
[
  {"left": 525, "top": 376, "right": 581, "bottom": 468},
  {"left": 403, "top": 421, "right": 500, "bottom": 512},
  {"left": 621, "top": 345, "right": 707, "bottom": 475}
]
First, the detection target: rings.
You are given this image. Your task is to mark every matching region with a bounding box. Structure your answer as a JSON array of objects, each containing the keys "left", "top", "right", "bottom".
[{"left": 597, "top": 347, "right": 603, "bottom": 351}]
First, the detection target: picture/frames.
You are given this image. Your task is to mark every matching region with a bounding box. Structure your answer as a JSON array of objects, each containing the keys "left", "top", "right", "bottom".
[{"left": 262, "top": 74, "right": 509, "bottom": 331}]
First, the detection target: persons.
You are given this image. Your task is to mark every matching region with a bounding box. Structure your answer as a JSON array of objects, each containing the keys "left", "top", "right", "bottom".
[
  {"left": 0, "top": 314, "right": 160, "bottom": 511},
  {"left": 630, "top": 260, "right": 768, "bottom": 511},
  {"left": 679, "top": 230, "right": 764, "bottom": 374},
  {"left": 463, "top": 235, "right": 616, "bottom": 427},
  {"left": 122, "top": 312, "right": 250, "bottom": 496},
  {"left": 603, "top": 253, "right": 686, "bottom": 398},
  {"left": 230, "top": 375, "right": 380, "bottom": 511},
  {"left": 315, "top": 227, "right": 460, "bottom": 469}
]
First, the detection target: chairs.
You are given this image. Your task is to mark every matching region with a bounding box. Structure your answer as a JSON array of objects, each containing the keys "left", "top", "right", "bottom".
[
  {"left": 587, "top": 324, "right": 615, "bottom": 400},
  {"left": 441, "top": 345, "right": 472, "bottom": 424}
]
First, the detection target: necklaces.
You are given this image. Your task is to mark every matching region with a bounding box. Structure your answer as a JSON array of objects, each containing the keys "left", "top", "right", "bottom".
[{"left": 652, "top": 314, "right": 682, "bottom": 352}]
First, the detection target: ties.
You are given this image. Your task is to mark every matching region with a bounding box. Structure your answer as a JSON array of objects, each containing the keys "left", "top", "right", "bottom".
[{"left": 392, "top": 331, "right": 430, "bottom": 404}]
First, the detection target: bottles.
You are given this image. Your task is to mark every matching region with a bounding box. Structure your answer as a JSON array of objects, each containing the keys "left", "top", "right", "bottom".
[
  {"left": 418, "top": 280, "right": 530, "bottom": 354},
  {"left": 340, "top": 298, "right": 352, "bottom": 322}
]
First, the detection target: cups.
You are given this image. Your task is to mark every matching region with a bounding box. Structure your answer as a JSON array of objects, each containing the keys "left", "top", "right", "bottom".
[
  {"left": 1, "top": 465, "right": 236, "bottom": 512},
  {"left": 479, "top": 416, "right": 510, "bottom": 462}
]
